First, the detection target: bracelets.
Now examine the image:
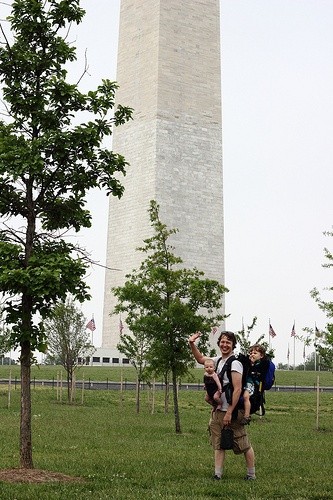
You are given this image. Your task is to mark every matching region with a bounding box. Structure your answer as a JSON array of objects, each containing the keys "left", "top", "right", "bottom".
[{"left": 219, "top": 389, "right": 221, "bottom": 390}]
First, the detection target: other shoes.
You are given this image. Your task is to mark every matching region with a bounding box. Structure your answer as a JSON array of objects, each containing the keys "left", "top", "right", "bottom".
[{"left": 241, "top": 416, "right": 251, "bottom": 425}]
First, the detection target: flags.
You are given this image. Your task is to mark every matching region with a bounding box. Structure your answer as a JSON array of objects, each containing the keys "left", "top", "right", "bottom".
[
  {"left": 269, "top": 324, "right": 276, "bottom": 338},
  {"left": 212, "top": 327, "right": 218, "bottom": 336},
  {"left": 291, "top": 325, "right": 295, "bottom": 337},
  {"left": 119, "top": 320, "right": 124, "bottom": 332},
  {"left": 86, "top": 318, "right": 96, "bottom": 331}
]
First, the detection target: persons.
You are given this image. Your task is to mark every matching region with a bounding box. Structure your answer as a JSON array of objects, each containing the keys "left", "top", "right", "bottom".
[
  {"left": 202, "top": 359, "right": 223, "bottom": 414},
  {"left": 188, "top": 330, "right": 256, "bottom": 482},
  {"left": 239, "top": 344, "right": 266, "bottom": 426}
]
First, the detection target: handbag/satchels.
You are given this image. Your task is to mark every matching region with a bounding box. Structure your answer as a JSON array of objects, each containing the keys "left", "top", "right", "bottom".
[{"left": 219, "top": 423, "right": 234, "bottom": 449}]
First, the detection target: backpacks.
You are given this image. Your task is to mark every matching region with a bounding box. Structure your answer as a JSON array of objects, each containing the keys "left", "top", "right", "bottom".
[
  {"left": 215, "top": 353, "right": 266, "bottom": 416},
  {"left": 260, "top": 354, "right": 276, "bottom": 391}
]
